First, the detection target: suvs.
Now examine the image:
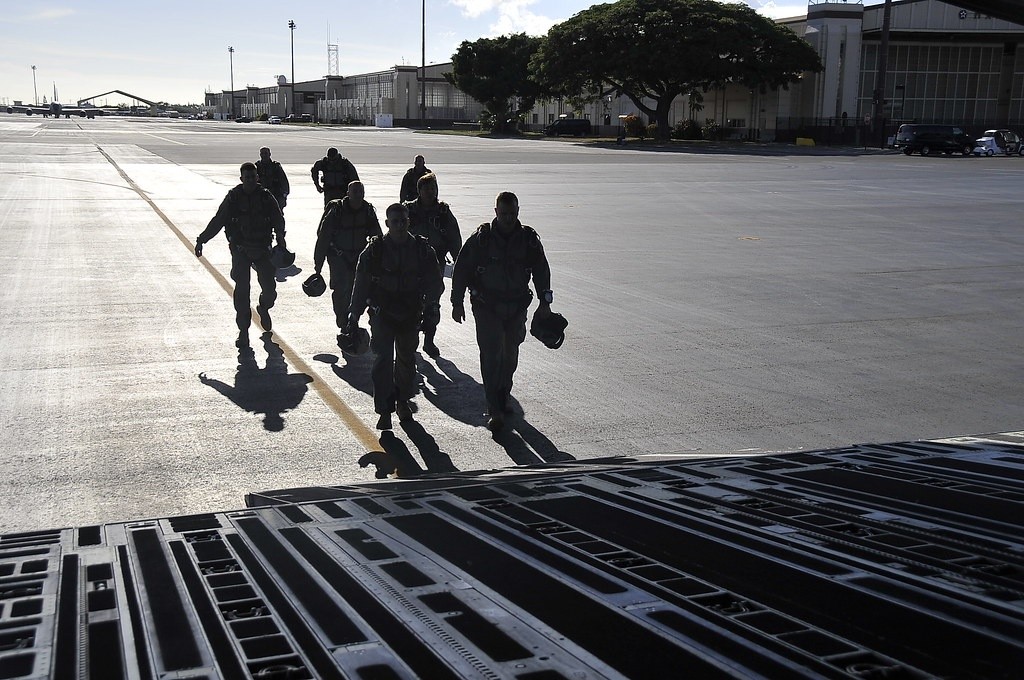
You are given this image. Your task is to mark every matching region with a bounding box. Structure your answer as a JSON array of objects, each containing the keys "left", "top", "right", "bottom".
[{"left": 894, "top": 124, "right": 977, "bottom": 157}]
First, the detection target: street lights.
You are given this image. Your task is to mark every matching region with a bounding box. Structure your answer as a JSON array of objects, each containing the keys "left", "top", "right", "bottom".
[
  {"left": 288, "top": 19, "right": 297, "bottom": 111},
  {"left": 31, "top": 65, "right": 38, "bottom": 107},
  {"left": 227, "top": 45, "right": 236, "bottom": 114}
]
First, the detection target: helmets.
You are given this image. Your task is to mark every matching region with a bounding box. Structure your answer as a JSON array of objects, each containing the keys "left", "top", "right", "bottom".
[
  {"left": 530, "top": 310, "right": 569, "bottom": 349},
  {"left": 338, "top": 328, "right": 370, "bottom": 357},
  {"left": 302, "top": 273, "right": 326, "bottom": 296},
  {"left": 269, "top": 245, "right": 295, "bottom": 268}
]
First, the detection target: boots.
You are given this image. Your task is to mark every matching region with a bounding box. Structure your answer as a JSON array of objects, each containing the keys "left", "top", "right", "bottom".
[
  {"left": 423, "top": 333, "right": 440, "bottom": 358},
  {"left": 498, "top": 382, "right": 514, "bottom": 414},
  {"left": 482, "top": 397, "right": 499, "bottom": 431}
]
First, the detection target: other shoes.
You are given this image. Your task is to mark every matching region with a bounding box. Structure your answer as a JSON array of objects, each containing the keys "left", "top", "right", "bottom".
[
  {"left": 337, "top": 316, "right": 348, "bottom": 328},
  {"left": 376, "top": 410, "right": 392, "bottom": 429},
  {"left": 236, "top": 329, "right": 249, "bottom": 347},
  {"left": 257, "top": 305, "right": 272, "bottom": 331},
  {"left": 396, "top": 399, "right": 411, "bottom": 420}
]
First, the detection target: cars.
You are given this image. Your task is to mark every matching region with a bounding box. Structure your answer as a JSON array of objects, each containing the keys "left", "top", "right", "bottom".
[
  {"left": 268, "top": 115, "right": 281, "bottom": 125},
  {"left": 973, "top": 129, "right": 1024, "bottom": 158}
]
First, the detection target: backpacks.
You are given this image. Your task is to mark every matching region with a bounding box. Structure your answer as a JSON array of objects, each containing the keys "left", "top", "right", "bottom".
[{"left": 317, "top": 200, "right": 370, "bottom": 243}]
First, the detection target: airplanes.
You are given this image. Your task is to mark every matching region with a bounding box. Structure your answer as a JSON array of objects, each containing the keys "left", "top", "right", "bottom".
[{"left": 0, "top": 101, "right": 110, "bottom": 118}]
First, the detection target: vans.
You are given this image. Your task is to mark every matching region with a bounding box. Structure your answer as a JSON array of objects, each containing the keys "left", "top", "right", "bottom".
[{"left": 541, "top": 119, "right": 592, "bottom": 138}]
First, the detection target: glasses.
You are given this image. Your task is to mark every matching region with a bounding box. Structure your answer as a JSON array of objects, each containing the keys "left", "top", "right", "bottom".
[{"left": 387, "top": 218, "right": 410, "bottom": 226}]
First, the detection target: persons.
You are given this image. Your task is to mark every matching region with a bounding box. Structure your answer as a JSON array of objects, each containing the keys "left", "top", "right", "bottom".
[
  {"left": 346, "top": 203, "right": 444, "bottom": 432},
  {"left": 396, "top": 154, "right": 431, "bottom": 204},
  {"left": 313, "top": 180, "right": 383, "bottom": 334},
  {"left": 195, "top": 163, "right": 286, "bottom": 348},
  {"left": 310, "top": 147, "right": 360, "bottom": 204},
  {"left": 402, "top": 172, "right": 461, "bottom": 353},
  {"left": 254, "top": 148, "right": 289, "bottom": 212},
  {"left": 448, "top": 190, "right": 554, "bottom": 431}
]
